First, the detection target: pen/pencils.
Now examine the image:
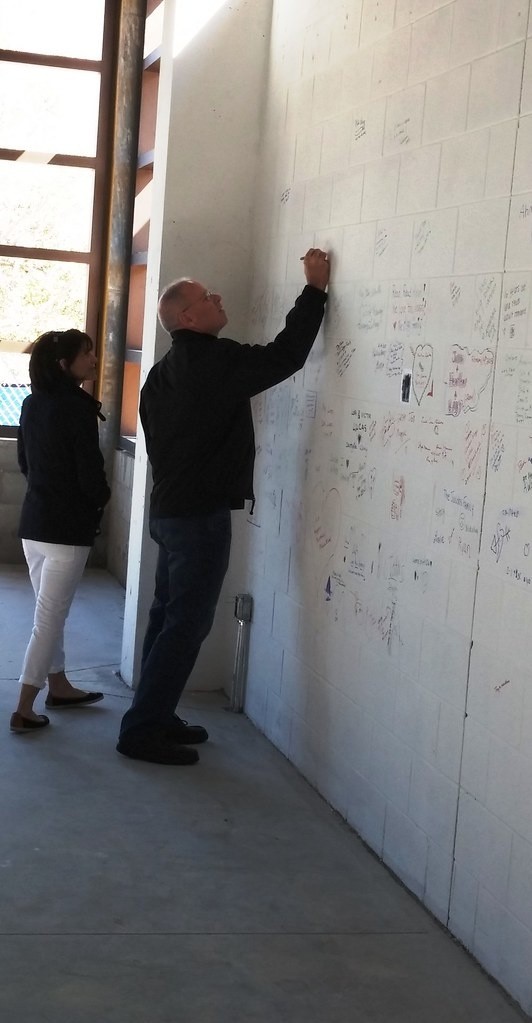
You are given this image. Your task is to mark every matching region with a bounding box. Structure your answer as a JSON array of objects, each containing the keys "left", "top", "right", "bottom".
[{"left": 300, "top": 257, "right": 304, "bottom": 260}]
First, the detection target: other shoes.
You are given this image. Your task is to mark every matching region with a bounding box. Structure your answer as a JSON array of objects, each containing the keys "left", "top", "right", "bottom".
[
  {"left": 10, "top": 712, "right": 51, "bottom": 732},
  {"left": 167, "top": 724, "right": 208, "bottom": 744},
  {"left": 45, "top": 689, "right": 104, "bottom": 710},
  {"left": 116, "top": 738, "right": 199, "bottom": 766}
]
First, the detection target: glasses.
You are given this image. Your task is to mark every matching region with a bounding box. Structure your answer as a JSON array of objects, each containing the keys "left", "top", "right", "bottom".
[{"left": 179, "top": 288, "right": 214, "bottom": 316}]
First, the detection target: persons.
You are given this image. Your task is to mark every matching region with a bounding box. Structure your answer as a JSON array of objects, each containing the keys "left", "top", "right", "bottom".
[
  {"left": 9, "top": 329, "right": 112, "bottom": 734},
  {"left": 116, "top": 248, "right": 331, "bottom": 766}
]
